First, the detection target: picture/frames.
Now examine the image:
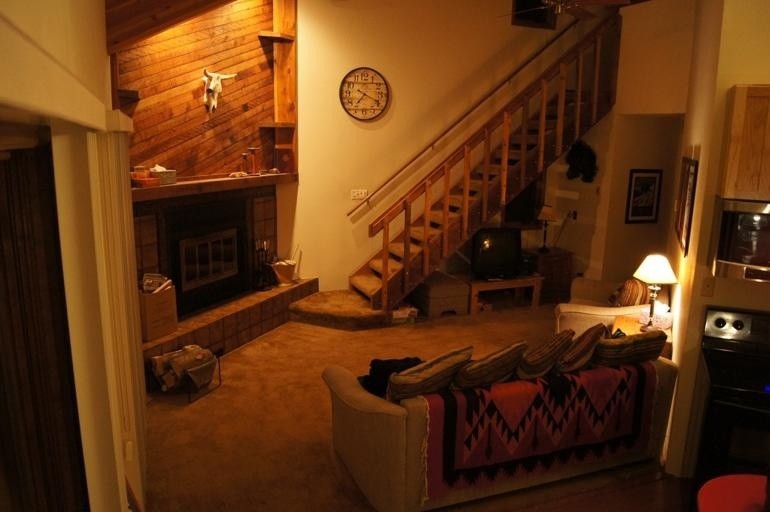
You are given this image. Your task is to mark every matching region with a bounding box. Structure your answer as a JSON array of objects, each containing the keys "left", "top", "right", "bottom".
[
  {"left": 624, "top": 168, "right": 663, "bottom": 223},
  {"left": 673, "top": 154, "right": 698, "bottom": 256}
]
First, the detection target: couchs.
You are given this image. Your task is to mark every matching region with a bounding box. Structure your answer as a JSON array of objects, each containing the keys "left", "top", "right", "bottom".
[{"left": 321, "top": 354, "right": 678, "bottom": 512}]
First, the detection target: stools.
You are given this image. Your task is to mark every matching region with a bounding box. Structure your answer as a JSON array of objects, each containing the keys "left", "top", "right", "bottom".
[{"left": 422, "top": 273, "right": 468, "bottom": 315}]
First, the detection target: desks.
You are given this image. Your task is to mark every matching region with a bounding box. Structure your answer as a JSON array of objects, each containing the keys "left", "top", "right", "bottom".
[{"left": 468, "top": 274, "right": 543, "bottom": 313}]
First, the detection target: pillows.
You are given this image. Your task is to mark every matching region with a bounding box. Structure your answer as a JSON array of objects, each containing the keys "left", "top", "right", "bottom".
[
  {"left": 608, "top": 279, "right": 648, "bottom": 307},
  {"left": 368, "top": 323, "right": 666, "bottom": 405}
]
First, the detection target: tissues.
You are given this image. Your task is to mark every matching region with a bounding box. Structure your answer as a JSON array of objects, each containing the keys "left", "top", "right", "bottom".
[{"left": 149, "top": 163, "right": 177, "bottom": 185}]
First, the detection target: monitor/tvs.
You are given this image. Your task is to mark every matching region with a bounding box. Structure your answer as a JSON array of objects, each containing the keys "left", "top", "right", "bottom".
[{"left": 471, "top": 227, "right": 521, "bottom": 276}]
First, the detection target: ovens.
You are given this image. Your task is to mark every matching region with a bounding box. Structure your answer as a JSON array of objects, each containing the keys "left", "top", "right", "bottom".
[{"left": 698, "top": 303, "right": 770, "bottom": 477}]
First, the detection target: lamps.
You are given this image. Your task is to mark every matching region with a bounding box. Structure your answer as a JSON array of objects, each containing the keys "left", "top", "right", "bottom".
[{"left": 631, "top": 254, "right": 678, "bottom": 332}]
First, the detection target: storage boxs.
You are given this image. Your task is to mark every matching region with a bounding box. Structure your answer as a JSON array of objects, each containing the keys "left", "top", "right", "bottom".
[{"left": 138, "top": 284, "right": 177, "bottom": 344}]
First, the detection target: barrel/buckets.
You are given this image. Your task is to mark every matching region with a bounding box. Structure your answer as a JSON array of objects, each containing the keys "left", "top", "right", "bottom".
[{"left": 268, "top": 257, "right": 297, "bottom": 287}]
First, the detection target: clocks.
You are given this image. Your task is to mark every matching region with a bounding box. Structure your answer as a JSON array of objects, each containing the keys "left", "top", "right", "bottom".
[{"left": 339, "top": 67, "right": 391, "bottom": 120}]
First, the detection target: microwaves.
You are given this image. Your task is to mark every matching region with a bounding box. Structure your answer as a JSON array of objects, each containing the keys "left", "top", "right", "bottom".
[{"left": 709, "top": 197, "right": 770, "bottom": 286}]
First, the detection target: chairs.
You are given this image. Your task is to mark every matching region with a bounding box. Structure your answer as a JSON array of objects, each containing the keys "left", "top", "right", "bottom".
[{"left": 553, "top": 277, "right": 670, "bottom": 341}]
